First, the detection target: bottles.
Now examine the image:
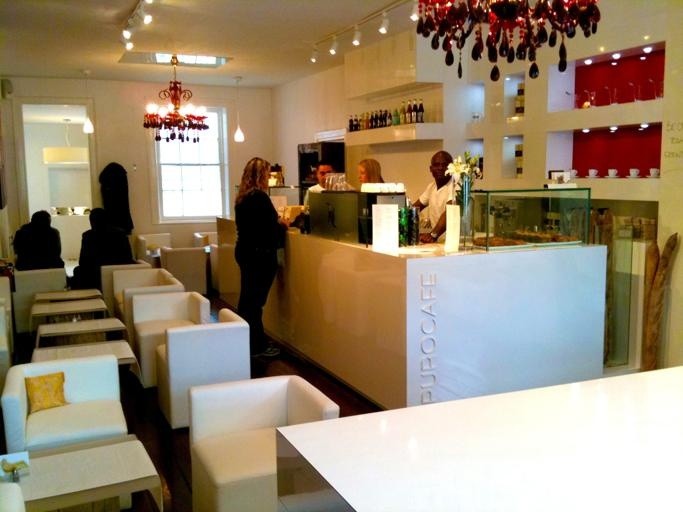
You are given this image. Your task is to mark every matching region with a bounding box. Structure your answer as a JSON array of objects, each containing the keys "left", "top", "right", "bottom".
[
  {"left": 476, "top": 157, "right": 483, "bottom": 179},
  {"left": 399, "top": 208, "right": 408, "bottom": 247},
  {"left": 348, "top": 98, "right": 425, "bottom": 132},
  {"left": 409, "top": 208, "right": 420, "bottom": 245}
]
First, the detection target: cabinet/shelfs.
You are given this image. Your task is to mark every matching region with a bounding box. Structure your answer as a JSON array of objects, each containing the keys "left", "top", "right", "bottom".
[{"left": 466, "top": 41, "right": 665, "bottom": 242}]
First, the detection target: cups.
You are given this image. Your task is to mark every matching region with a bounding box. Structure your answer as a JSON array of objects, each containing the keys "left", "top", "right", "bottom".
[
  {"left": 566, "top": 83, "right": 657, "bottom": 110},
  {"left": 324, "top": 173, "right": 348, "bottom": 191},
  {"left": 472, "top": 112, "right": 482, "bottom": 121},
  {"left": 568, "top": 168, "right": 660, "bottom": 177},
  {"left": 360, "top": 182, "right": 405, "bottom": 193}
]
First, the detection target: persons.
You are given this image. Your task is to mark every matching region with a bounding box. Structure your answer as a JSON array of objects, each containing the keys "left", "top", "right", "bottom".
[
  {"left": 72, "top": 208, "right": 109, "bottom": 290},
  {"left": 411, "top": 150, "right": 461, "bottom": 243},
  {"left": 356, "top": 159, "right": 386, "bottom": 184},
  {"left": 233, "top": 156, "right": 280, "bottom": 357},
  {"left": 303, "top": 159, "right": 354, "bottom": 205},
  {"left": 11, "top": 210, "right": 65, "bottom": 272}
]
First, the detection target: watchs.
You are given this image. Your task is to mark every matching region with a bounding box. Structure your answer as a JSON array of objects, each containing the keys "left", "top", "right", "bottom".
[{"left": 430, "top": 229, "right": 438, "bottom": 242}]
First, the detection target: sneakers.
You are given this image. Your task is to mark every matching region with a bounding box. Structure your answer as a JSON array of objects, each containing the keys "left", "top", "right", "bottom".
[{"left": 251, "top": 347, "right": 280, "bottom": 357}]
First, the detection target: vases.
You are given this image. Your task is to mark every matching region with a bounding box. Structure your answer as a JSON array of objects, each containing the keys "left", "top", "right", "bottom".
[{"left": 457, "top": 198, "right": 473, "bottom": 251}]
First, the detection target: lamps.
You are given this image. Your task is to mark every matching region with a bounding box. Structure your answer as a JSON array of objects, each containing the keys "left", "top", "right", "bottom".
[
  {"left": 379, "top": 9, "right": 388, "bottom": 34},
  {"left": 233, "top": 75, "right": 245, "bottom": 143},
  {"left": 43, "top": 117, "right": 90, "bottom": 165},
  {"left": 309, "top": 40, "right": 319, "bottom": 63},
  {"left": 81, "top": 69, "right": 97, "bottom": 135},
  {"left": 329, "top": 34, "right": 339, "bottom": 56},
  {"left": 411, "top": 1, "right": 417, "bottom": 22},
  {"left": 417, "top": 0, "right": 599, "bottom": 82},
  {"left": 122, "top": 7, "right": 154, "bottom": 53},
  {"left": 352, "top": 22, "right": 362, "bottom": 46},
  {"left": 143, "top": 54, "right": 208, "bottom": 143}
]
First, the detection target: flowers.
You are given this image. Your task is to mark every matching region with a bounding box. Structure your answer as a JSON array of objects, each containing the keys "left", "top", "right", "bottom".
[{"left": 445, "top": 151, "right": 481, "bottom": 216}]
